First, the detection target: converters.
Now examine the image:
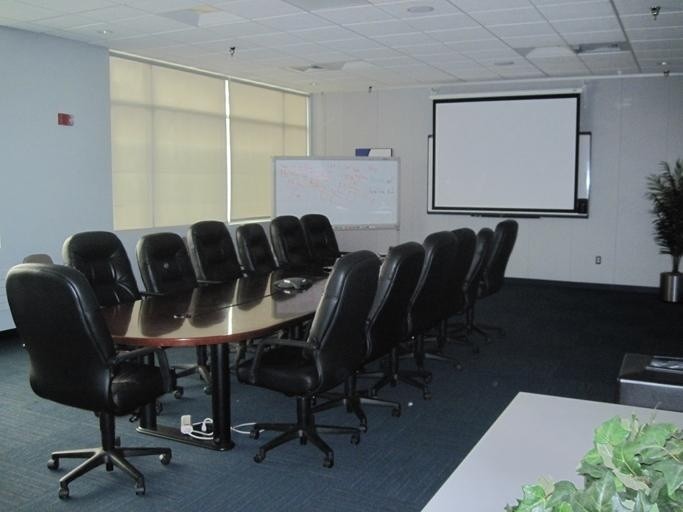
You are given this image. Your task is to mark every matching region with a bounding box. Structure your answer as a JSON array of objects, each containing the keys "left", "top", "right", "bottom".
[{"left": 181, "top": 415, "right": 193, "bottom": 434}]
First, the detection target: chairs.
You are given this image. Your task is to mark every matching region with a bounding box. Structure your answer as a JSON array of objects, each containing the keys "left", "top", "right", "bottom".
[
  {"left": 408, "top": 216, "right": 518, "bottom": 398},
  {"left": 318, "top": 241, "right": 424, "bottom": 431},
  {"left": 61, "top": 227, "right": 184, "bottom": 424},
  {"left": 249, "top": 248, "right": 381, "bottom": 470},
  {"left": 5, "top": 262, "right": 179, "bottom": 499},
  {"left": 134, "top": 230, "right": 213, "bottom": 396},
  {"left": 299, "top": 214, "right": 365, "bottom": 328},
  {"left": 268, "top": 216, "right": 331, "bottom": 358},
  {"left": 234, "top": 223, "right": 299, "bottom": 370},
  {"left": 186, "top": 220, "right": 261, "bottom": 378}
]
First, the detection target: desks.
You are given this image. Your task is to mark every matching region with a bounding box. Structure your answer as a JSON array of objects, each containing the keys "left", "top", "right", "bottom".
[
  {"left": 617, "top": 351, "right": 683, "bottom": 413},
  {"left": 422, "top": 391, "right": 683, "bottom": 512},
  {"left": 106, "top": 277, "right": 392, "bottom": 450}
]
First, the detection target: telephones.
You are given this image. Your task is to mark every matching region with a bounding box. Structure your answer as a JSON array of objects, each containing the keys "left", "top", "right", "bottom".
[{"left": 274, "top": 277, "right": 313, "bottom": 289}]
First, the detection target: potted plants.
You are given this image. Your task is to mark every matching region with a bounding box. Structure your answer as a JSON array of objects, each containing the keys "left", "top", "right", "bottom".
[{"left": 641, "top": 157, "right": 683, "bottom": 302}]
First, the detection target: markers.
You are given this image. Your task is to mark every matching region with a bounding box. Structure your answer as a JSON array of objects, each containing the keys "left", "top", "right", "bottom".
[{"left": 340, "top": 225, "right": 377, "bottom": 229}]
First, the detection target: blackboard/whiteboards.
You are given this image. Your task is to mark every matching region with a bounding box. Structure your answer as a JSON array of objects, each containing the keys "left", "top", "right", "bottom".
[
  {"left": 272, "top": 155, "right": 401, "bottom": 232},
  {"left": 427, "top": 131, "right": 592, "bottom": 218}
]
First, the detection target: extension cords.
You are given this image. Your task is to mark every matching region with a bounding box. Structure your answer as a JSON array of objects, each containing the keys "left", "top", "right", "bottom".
[{"left": 193, "top": 422, "right": 212, "bottom": 436}]
